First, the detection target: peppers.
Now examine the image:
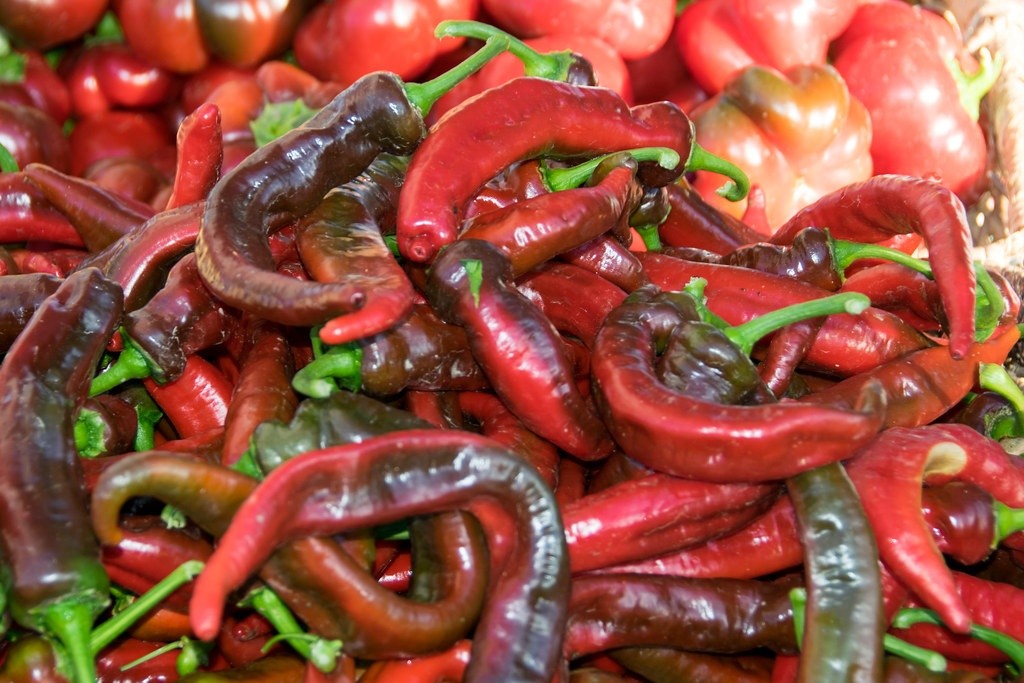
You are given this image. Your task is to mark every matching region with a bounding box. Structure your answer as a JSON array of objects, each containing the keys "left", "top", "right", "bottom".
[{"left": 1, "top": 0, "right": 1019, "bottom": 683}]
